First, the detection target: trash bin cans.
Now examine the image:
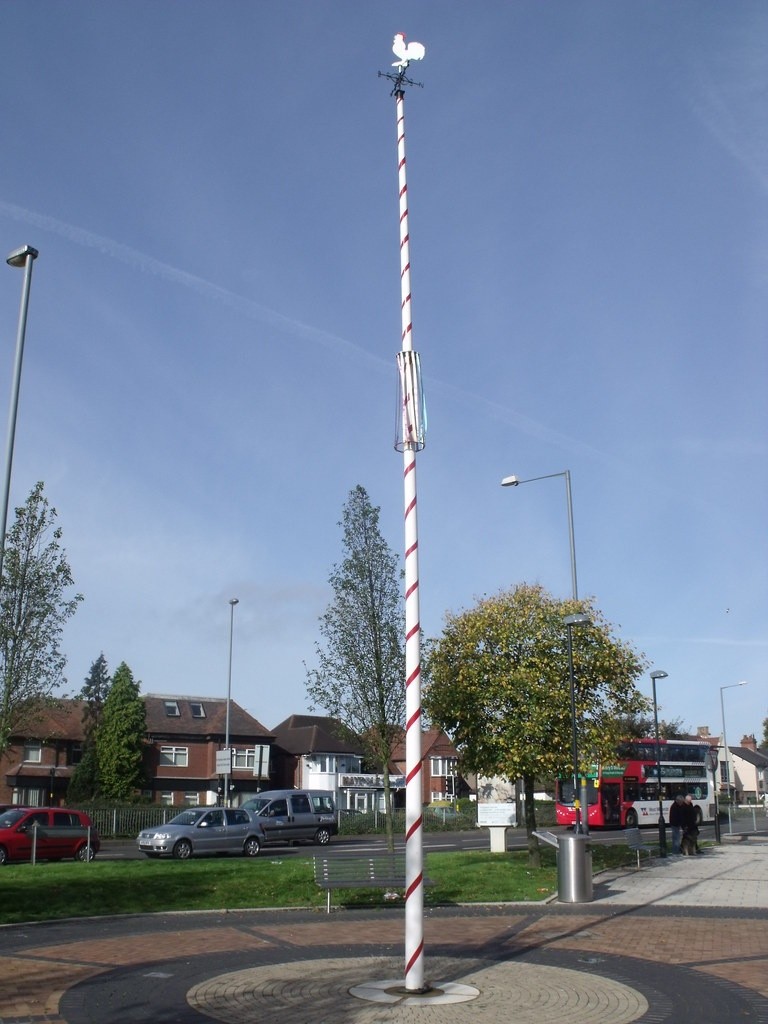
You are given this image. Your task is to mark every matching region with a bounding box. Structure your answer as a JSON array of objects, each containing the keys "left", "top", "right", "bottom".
[{"left": 556, "top": 834, "right": 594, "bottom": 903}]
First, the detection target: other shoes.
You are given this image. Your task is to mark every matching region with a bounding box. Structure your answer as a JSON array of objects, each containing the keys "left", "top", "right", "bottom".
[{"left": 695, "top": 850, "right": 703, "bottom": 854}]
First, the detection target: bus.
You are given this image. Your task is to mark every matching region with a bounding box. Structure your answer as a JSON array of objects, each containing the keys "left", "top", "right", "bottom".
[{"left": 555, "top": 737, "right": 720, "bottom": 829}]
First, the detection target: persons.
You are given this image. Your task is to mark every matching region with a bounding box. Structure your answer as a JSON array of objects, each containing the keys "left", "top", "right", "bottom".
[
  {"left": 204, "top": 814, "right": 213, "bottom": 827},
  {"left": 265, "top": 804, "right": 283, "bottom": 817},
  {"left": 670, "top": 795, "right": 700, "bottom": 855}
]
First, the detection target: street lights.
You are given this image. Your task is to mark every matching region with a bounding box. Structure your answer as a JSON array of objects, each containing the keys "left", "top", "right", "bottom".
[
  {"left": 224, "top": 598, "right": 239, "bottom": 808},
  {"left": 563, "top": 614, "right": 590, "bottom": 834},
  {"left": 650, "top": 669, "right": 668, "bottom": 828},
  {"left": 0, "top": 245, "right": 38, "bottom": 584},
  {"left": 720, "top": 681, "right": 748, "bottom": 808},
  {"left": 502, "top": 470, "right": 585, "bottom": 732}
]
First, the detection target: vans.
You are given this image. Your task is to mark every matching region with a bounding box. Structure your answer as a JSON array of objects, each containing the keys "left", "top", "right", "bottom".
[{"left": 236, "top": 790, "right": 338, "bottom": 846}]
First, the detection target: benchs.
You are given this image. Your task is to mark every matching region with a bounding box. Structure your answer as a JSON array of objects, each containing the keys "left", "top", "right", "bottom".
[
  {"left": 313, "top": 847, "right": 435, "bottom": 914},
  {"left": 623, "top": 827, "right": 661, "bottom": 869}
]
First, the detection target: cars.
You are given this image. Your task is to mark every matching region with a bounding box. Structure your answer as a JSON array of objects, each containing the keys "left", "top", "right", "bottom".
[
  {"left": 136, "top": 808, "right": 266, "bottom": 860},
  {"left": 0, "top": 804, "right": 101, "bottom": 865}
]
